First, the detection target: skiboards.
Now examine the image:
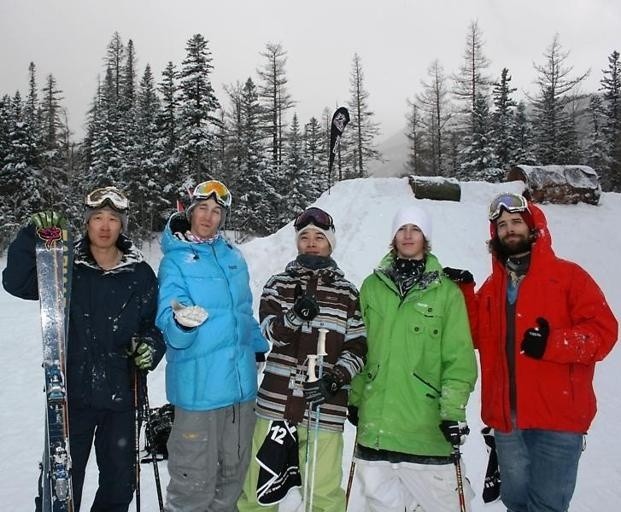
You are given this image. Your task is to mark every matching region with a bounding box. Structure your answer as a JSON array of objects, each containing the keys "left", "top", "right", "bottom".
[{"left": 34, "top": 218, "right": 75, "bottom": 512}]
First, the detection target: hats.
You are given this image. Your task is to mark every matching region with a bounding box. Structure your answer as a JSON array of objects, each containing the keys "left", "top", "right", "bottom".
[
  {"left": 185, "top": 186, "right": 227, "bottom": 230},
  {"left": 83, "top": 189, "right": 132, "bottom": 230},
  {"left": 391, "top": 208, "right": 433, "bottom": 242},
  {"left": 520, "top": 210, "right": 535, "bottom": 229},
  {"left": 294, "top": 213, "right": 337, "bottom": 252}
]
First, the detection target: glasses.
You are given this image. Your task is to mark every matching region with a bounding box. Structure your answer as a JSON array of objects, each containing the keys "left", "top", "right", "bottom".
[
  {"left": 489, "top": 192, "right": 532, "bottom": 221},
  {"left": 86, "top": 186, "right": 128, "bottom": 210},
  {"left": 193, "top": 180, "right": 233, "bottom": 207},
  {"left": 294, "top": 208, "right": 332, "bottom": 231}
]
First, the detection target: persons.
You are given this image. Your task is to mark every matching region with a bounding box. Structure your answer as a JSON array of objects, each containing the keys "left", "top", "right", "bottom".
[
  {"left": 2, "top": 186, "right": 168, "bottom": 511},
  {"left": 440, "top": 191, "right": 620, "bottom": 511},
  {"left": 234, "top": 206, "right": 369, "bottom": 512},
  {"left": 345, "top": 203, "right": 477, "bottom": 511},
  {"left": 156, "top": 179, "right": 270, "bottom": 512}
]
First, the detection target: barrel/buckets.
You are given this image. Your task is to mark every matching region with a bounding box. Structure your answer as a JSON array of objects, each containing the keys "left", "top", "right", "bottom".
[
  {"left": 507, "top": 163, "right": 601, "bottom": 207},
  {"left": 401, "top": 172, "right": 461, "bottom": 201}
]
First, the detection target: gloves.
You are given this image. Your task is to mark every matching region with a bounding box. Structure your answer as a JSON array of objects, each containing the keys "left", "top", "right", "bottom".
[
  {"left": 286, "top": 296, "right": 319, "bottom": 330},
  {"left": 170, "top": 301, "right": 210, "bottom": 328},
  {"left": 303, "top": 366, "right": 352, "bottom": 407},
  {"left": 18, "top": 212, "right": 61, "bottom": 253},
  {"left": 347, "top": 406, "right": 360, "bottom": 424},
  {"left": 132, "top": 334, "right": 162, "bottom": 369},
  {"left": 441, "top": 420, "right": 469, "bottom": 446},
  {"left": 520, "top": 318, "right": 550, "bottom": 359}
]
